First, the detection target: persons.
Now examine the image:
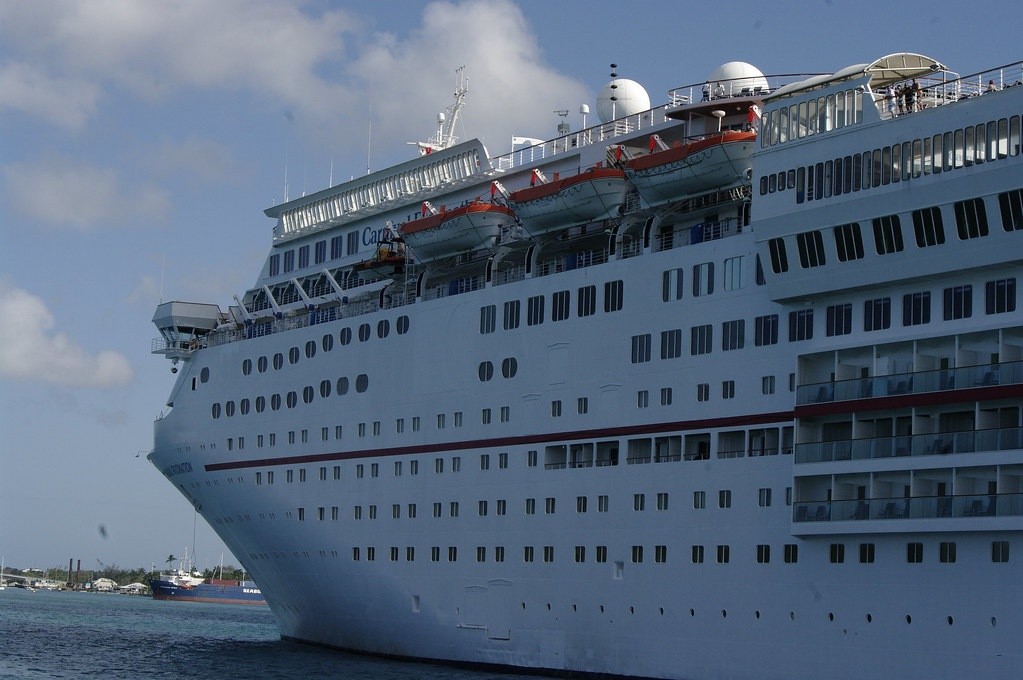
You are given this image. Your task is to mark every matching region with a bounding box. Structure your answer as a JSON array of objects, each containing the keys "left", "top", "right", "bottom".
[
  {"left": 902, "top": 82, "right": 913, "bottom": 113},
  {"left": 988, "top": 80, "right": 996, "bottom": 91},
  {"left": 894, "top": 85, "right": 903, "bottom": 116},
  {"left": 714, "top": 81, "right": 724, "bottom": 99},
  {"left": 702, "top": 80, "right": 711, "bottom": 101},
  {"left": 882, "top": 86, "right": 896, "bottom": 118},
  {"left": 911, "top": 78, "right": 924, "bottom": 111}
]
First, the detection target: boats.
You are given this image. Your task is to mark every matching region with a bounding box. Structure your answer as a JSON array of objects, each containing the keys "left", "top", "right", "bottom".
[
  {"left": 148, "top": 554, "right": 268, "bottom": 604},
  {"left": 404, "top": 128, "right": 753, "bottom": 263}
]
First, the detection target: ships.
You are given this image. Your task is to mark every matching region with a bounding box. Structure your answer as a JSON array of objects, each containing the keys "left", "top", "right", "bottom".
[{"left": 145, "top": 52, "right": 1022, "bottom": 680}]
